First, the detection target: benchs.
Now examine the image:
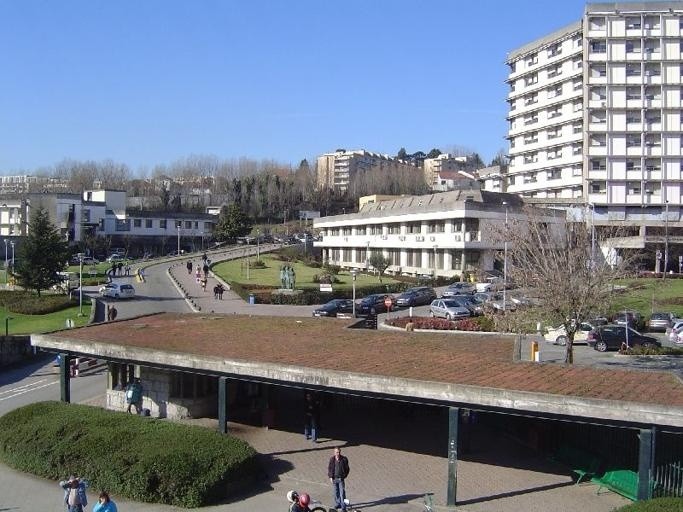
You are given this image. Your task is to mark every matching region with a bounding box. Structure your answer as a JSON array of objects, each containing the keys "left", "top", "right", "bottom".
[
  {"left": 569, "top": 457, "right": 596, "bottom": 484},
  {"left": 591, "top": 465, "right": 658, "bottom": 502}
]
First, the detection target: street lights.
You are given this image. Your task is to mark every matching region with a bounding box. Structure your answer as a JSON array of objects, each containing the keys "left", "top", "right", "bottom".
[
  {"left": 503, "top": 202, "right": 508, "bottom": 309},
  {"left": 257, "top": 229, "right": 259, "bottom": 262},
  {"left": 78, "top": 253, "right": 85, "bottom": 317},
  {"left": 4, "top": 239, "right": 9, "bottom": 284},
  {"left": 590, "top": 203, "right": 597, "bottom": 280},
  {"left": 201, "top": 232, "right": 204, "bottom": 251},
  {"left": 10, "top": 241, "right": 15, "bottom": 275},
  {"left": 350, "top": 270, "right": 359, "bottom": 318},
  {"left": 665, "top": 200, "right": 670, "bottom": 274},
  {"left": 177, "top": 226, "right": 182, "bottom": 256},
  {"left": 246, "top": 238, "right": 250, "bottom": 278}
]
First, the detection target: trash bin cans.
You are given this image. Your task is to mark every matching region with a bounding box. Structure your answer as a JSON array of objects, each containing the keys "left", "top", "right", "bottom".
[
  {"left": 248, "top": 294, "right": 255, "bottom": 305},
  {"left": 530, "top": 340, "right": 537, "bottom": 361}
]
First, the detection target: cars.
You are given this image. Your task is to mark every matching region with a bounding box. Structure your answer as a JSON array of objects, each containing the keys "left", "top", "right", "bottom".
[
  {"left": 362, "top": 294, "right": 398, "bottom": 315},
  {"left": 430, "top": 277, "right": 517, "bottom": 321},
  {"left": 99, "top": 282, "right": 134, "bottom": 299},
  {"left": 312, "top": 299, "right": 362, "bottom": 317},
  {"left": 109, "top": 248, "right": 126, "bottom": 254},
  {"left": 397, "top": 287, "right": 437, "bottom": 307},
  {"left": 105, "top": 254, "right": 124, "bottom": 262},
  {"left": 71, "top": 256, "right": 80, "bottom": 264},
  {"left": 544, "top": 314, "right": 683, "bottom": 351},
  {"left": 285, "top": 233, "right": 318, "bottom": 244},
  {"left": 83, "top": 257, "right": 99, "bottom": 265}
]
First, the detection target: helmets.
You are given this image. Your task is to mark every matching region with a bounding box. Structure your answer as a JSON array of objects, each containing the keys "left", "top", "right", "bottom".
[{"left": 299, "top": 493, "right": 310, "bottom": 508}]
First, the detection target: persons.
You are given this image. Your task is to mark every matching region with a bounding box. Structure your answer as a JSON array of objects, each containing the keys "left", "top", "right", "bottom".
[
  {"left": 187, "top": 252, "right": 225, "bottom": 300},
  {"left": 111, "top": 261, "right": 131, "bottom": 276},
  {"left": 63, "top": 481, "right": 87, "bottom": 512},
  {"left": 96, "top": 492, "right": 117, "bottom": 512},
  {"left": 303, "top": 391, "right": 320, "bottom": 440},
  {"left": 406, "top": 319, "right": 414, "bottom": 331},
  {"left": 329, "top": 446, "right": 349, "bottom": 512},
  {"left": 107, "top": 305, "right": 117, "bottom": 321},
  {"left": 127, "top": 377, "right": 143, "bottom": 414}
]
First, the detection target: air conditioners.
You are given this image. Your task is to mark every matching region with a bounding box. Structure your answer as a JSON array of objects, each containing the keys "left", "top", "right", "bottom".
[{"left": 344, "top": 235, "right": 461, "bottom": 242}]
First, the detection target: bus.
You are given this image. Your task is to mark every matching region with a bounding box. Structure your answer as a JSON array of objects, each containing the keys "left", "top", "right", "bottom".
[{"left": 48, "top": 272, "right": 78, "bottom": 293}]
